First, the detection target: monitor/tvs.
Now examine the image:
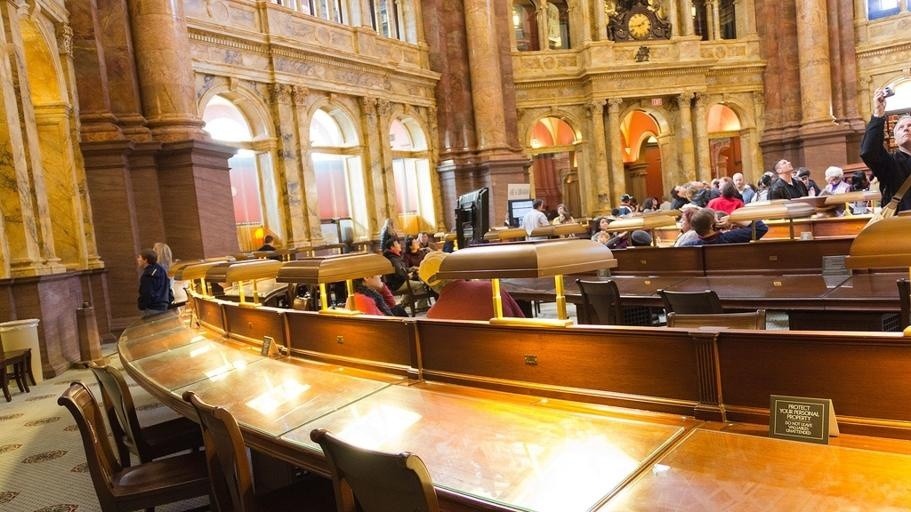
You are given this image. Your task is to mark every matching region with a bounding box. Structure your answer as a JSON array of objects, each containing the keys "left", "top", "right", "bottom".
[
  {"left": 508, "top": 198, "right": 534, "bottom": 227},
  {"left": 454, "top": 186, "right": 489, "bottom": 248}
]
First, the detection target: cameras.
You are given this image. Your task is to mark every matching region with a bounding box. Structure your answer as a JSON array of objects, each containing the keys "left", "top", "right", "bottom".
[{"left": 882, "top": 83, "right": 895, "bottom": 97}]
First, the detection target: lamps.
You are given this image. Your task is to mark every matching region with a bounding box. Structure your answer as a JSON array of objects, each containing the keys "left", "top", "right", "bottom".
[
  {"left": 205, "top": 259, "right": 283, "bottom": 302},
  {"left": 824, "top": 191, "right": 882, "bottom": 218},
  {"left": 294, "top": 243, "right": 348, "bottom": 253},
  {"left": 275, "top": 253, "right": 396, "bottom": 316},
  {"left": 844, "top": 212, "right": 910, "bottom": 270},
  {"left": 235, "top": 250, "right": 295, "bottom": 261},
  {"left": 728, "top": 198, "right": 817, "bottom": 242},
  {"left": 169, "top": 255, "right": 236, "bottom": 298},
  {"left": 434, "top": 231, "right": 457, "bottom": 248},
  {"left": 483, "top": 223, "right": 587, "bottom": 242},
  {"left": 606, "top": 214, "right": 676, "bottom": 248},
  {"left": 433, "top": 238, "right": 617, "bottom": 330},
  {"left": 351, "top": 239, "right": 381, "bottom": 252}
]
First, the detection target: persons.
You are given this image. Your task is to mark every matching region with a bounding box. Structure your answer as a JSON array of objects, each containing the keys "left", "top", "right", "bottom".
[
  {"left": 135, "top": 247, "right": 173, "bottom": 318},
  {"left": 259, "top": 236, "right": 281, "bottom": 260},
  {"left": 859, "top": 85, "right": 911, "bottom": 212},
  {"left": 352, "top": 217, "right": 437, "bottom": 318},
  {"left": 152, "top": 242, "right": 188, "bottom": 307}
]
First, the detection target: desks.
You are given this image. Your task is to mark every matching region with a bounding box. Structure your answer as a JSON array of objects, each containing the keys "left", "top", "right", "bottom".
[
  {"left": 116, "top": 301, "right": 911, "bottom": 511},
  {"left": 467, "top": 235, "right": 911, "bottom": 331}
]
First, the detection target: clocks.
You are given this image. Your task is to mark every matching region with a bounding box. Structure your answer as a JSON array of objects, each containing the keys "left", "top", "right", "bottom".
[{"left": 627, "top": 12, "right": 652, "bottom": 41}]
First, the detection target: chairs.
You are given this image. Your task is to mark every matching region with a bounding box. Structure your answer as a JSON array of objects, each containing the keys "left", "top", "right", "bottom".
[
  {"left": 182, "top": 392, "right": 259, "bottom": 510},
  {"left": 309, "top": 429, "right": 441, "bottom": 511},
  {"left": 0, "top": 334, "right": 37, "bottom": 402},
  {"left": 57, "top": 380, "right": 235, "bottom": 511},
  {"left": 87, "top": 363, "right": 204, "bottom": 511}
]
